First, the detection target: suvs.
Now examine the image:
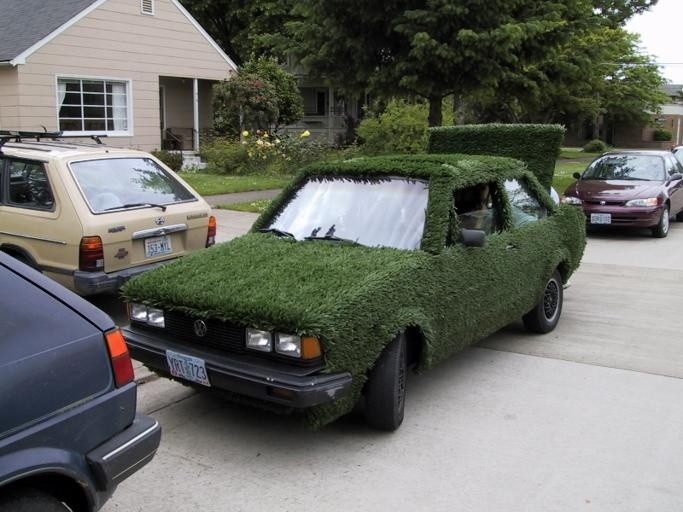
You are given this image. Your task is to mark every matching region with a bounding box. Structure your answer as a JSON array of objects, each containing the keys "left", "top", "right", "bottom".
[{"left": 0, "top": 127, "right": 220, "bottom": 307}]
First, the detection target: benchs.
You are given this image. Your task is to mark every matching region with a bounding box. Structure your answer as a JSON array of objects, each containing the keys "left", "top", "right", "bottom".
[{"left": 166, "top": 127, "right": 204, "bottom": 150}]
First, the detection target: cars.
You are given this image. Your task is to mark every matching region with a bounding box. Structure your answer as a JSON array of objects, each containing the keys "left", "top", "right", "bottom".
[
  {"left": 0, "top": 250, "right": 163, "bottom": 510},
  {"left": 116, "top": 124, "right": 587, "bottom": 433},
  {"left": 671, "top": 146, "right": 683, "bottom": 166},
  {"left": 561, "top": 146, "right": 683, "bottom": 243}
]
